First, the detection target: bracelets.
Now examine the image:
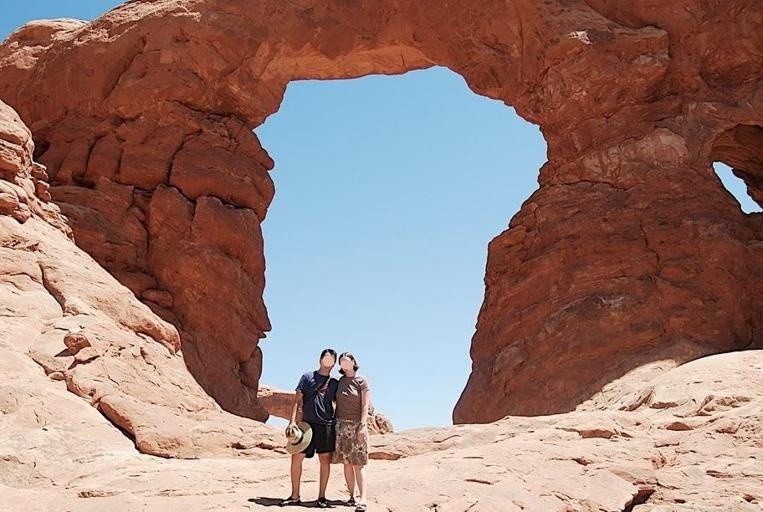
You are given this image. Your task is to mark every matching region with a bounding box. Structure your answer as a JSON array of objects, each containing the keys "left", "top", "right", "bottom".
[{"left": 359, "top": 422, "right": 367, "bottom": 426}]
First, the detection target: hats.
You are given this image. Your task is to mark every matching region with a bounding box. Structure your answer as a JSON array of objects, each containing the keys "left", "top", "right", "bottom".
[{"left": 285, "top": 422, "right": 312, "bottom": 456}]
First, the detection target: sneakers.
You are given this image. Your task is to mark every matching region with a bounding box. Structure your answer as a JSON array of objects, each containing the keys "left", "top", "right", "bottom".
[
  {"left": 354, "top": 504, "right": 366, "bottom": 511},
  {"left": 279, "top": 495, "right": 300, "bottom": 507},
  {"left": 343, "top": 498, "right": 356, "bottom": 505},
  {"left": 317, "top": 496, "right": 331, "bottom": 507}
]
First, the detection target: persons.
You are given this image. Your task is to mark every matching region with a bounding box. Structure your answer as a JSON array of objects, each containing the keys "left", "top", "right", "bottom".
[
  {"left": 278, "top": 349, "right": 340, "bottom": 508},
  {"left": 333, "top": 351, "right": 371, "bottom": 512}
]
